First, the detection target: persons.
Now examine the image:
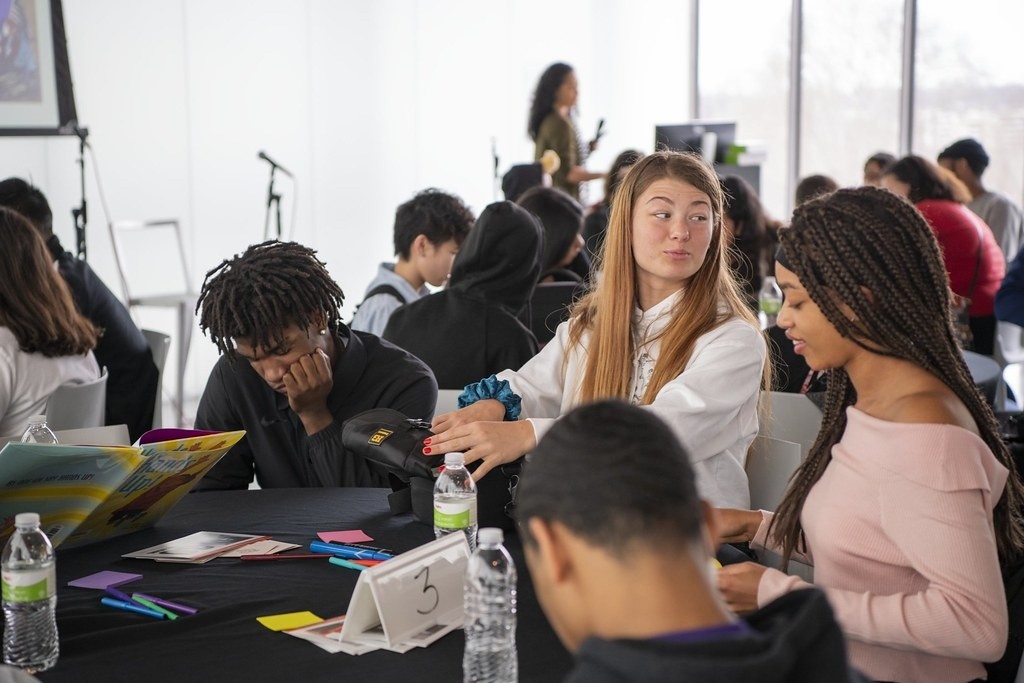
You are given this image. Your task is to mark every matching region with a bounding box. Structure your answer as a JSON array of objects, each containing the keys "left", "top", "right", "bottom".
[
  {"left": 514, "top": 399, "right": 855, "bottom": 683},
  {"left": 190, "top": 240, "right": 439, "bottom": 491},
  {"left": 865, "top": 151, "right": 895, "bottom": 187},
  {"left": 937, "top": 139, "right": 1024, "bottom": 262},
  {"left": 719, "top": 175, "right": 782, "bottom": 299},
  {"left": 796, "top": 175, "right": 837, "bottom": 207},
  {"left": 992, "top": 248, "right": 1024, "bottom": 366},
  {"left": 1, "top": 177, "right": 157, "bottom": 435},
  {"left": 381, "top": 199, "right": 544, "bottom": 389},
  {"left": 0, "top": 209, "right": 102, "bottom": 436},
  {"left": 352, "top": 186, "right": 474, "bottom": 339},
  {"left": 501, "top": 151, "right": 646, "bottom": 344},
  {"left": 422, "top": 148, "right": 773, "bottom": 561},
  {"left": 529, "top": 64, "right": 607, "bottom": 200},
  {"left": 883, "top": 155, "right": 1003, "bottom": 358},
  {"left": 713, "top": 186, "right": 1024, "bottom": 683}
]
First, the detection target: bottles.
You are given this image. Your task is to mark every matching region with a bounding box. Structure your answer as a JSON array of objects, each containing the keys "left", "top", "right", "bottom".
[
  {"left": 18, "top": 415, "right": 58, "bottom": 446},
  {"left": 757, "top": 276, "right": 784, "bottom": 330},
  {"left": 1, "top": 512, "right": 60, "bottom": 674},
  {"left": 461, "top": 527, "right": 518, "bottom": 683},
  {"left": 433, "top": 451, "right": 477, "bottom": 552}
]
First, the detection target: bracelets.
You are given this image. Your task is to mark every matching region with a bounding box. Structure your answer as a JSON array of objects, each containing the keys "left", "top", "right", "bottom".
[{"left": 457, "top": 374, "right": 521, "bottom": 421}]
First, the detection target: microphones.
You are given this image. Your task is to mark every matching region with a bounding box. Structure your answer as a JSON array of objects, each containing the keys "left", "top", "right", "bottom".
[
  {"left": 68, "top": 120, "right": 90, "bottom": 148},
  {"left": 258, "top": 152, "right": 283, "bottom": 172}
]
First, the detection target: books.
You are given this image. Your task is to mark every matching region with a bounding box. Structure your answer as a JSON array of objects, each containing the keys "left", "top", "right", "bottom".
[{"left": 0, "top": 427, "right": 246, "bottom": 553}]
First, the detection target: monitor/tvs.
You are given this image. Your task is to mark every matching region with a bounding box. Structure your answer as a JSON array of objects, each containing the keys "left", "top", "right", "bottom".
[{"left": 654, "top": 123, "right": 737, "bottom": 164}]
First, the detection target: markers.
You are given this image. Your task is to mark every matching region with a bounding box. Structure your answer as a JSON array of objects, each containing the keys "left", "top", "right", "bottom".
[
  {"left": 310, "top": 540, "right": 394, "bottom": 561},
  {"left": 329, "top": 540, "right": 393, "bottom": 555}
]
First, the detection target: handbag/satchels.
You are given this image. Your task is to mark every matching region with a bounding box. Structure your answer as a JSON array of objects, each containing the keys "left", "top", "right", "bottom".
[
  {"left": 949, "top": 288, "right": 974, "bottom": 348},
  {"left": 339, "top": 408, "right": 518, "bottom": 528}
]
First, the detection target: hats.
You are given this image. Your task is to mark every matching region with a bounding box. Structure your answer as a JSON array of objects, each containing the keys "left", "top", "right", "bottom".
[{"left": 501, "top": 149, "right": 560, "bottom": 203}]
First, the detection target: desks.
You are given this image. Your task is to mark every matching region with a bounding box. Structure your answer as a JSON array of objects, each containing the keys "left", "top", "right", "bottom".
[{"left": 0, "top": 484, "right": 760, "bottom": 683}]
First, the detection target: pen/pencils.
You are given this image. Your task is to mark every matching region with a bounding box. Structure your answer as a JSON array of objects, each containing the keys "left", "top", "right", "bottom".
[
  {"left": 132, "top": 596, "right": 179, "bottom": 621},
  {"left": 101, "top": 598, "right": 166, "bottom": 618},
  {"left": 348, "top": 559, "right": 382, "bottom": 567},
  {"left": 106, "top": 586, "right": 149, "bottom": 609},
  {"left": 132, "top": 593, "right": 198, "bottom": 614},
  {"left": 240, "top": 554, "right": 333, "bottom": 560},
  {"left": 330, "top": 557, "right": 383, "bottom": 570}
]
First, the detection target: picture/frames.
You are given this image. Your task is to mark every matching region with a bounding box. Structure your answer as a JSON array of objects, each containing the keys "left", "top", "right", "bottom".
[{"left": 0, "top": 0, "right": 80, "bottom": 137}]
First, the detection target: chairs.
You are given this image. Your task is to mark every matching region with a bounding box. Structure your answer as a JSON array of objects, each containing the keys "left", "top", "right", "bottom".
[
  {"left": 139, "top": 327, "right": 172, "bottom": 432},
  {"left": 420, "top": 277, "right": 1022, "bottom": 582},
  {"left": 45, "top": 364, "right": 110, "bottom": 433}
]
